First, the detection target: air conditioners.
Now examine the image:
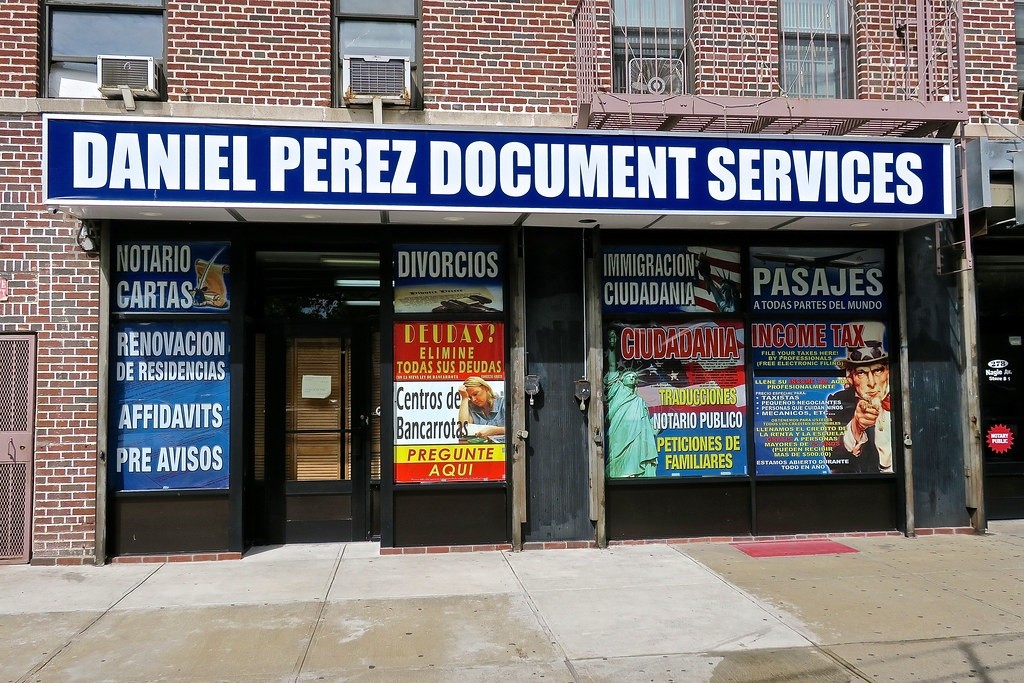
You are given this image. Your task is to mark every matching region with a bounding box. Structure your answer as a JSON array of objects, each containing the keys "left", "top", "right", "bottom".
[
  {"left": 343, "top": 54, "right": 411, "bottom": 106},
  {"left": 97, "top": 55, "right": 161, "bottom": 98}
]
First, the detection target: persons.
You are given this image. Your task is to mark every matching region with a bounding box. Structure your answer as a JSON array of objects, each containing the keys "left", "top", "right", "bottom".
[
  {"left": 696, "top": 251, "right": 742, "bottom": 313},
  {"left": 603, "top": 328, "right": 660, "bottom": 478},
  {"left": 824, "top": 322, "right": 894, "bottom": 474},
  {"left": 458, "top": 376, "right": 506, "bottom": 440}
]
